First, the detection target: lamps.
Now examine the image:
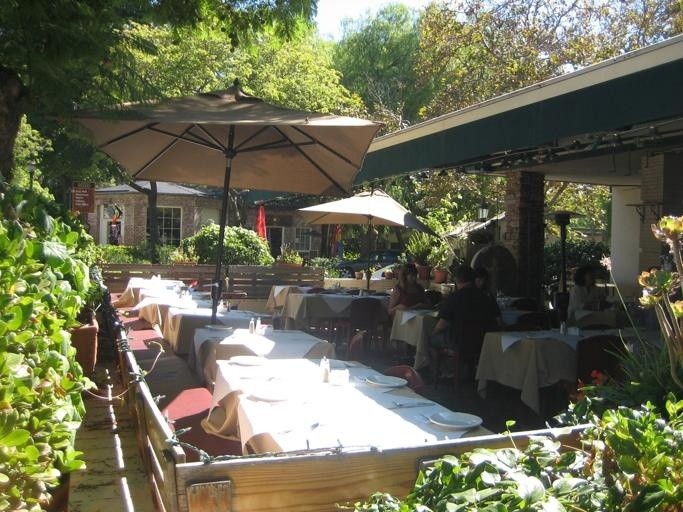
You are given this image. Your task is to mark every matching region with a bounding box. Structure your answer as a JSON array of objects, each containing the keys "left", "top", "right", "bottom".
[
  {"left": 352, "top": 169, "right": 448, "bottom": 192},
  {"left": 499, "top": 148, "right": 557, "bottom": 167},
  {"left": 477, "top": 161, "right": 489, "bottom": 222}
]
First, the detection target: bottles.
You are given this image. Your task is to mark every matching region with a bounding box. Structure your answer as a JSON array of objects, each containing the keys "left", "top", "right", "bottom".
[
  {"left": 226, "top": 299, "right": 232, "bottom": 312},
  {"left": 560, "top": 321, "right": 568, "bottom": 335},
  {"left": 495, "top": 296, "right": 512, "bottom": 311},
  {"left": 249, "top": 315, "right": 262, "bottom": 335},
  {"left": 271, "top": 304, "right": 287, "bottom": 330},
  {"left": 332, "top": 282, "right": 341, "bottom": 289},
  {"left": 318, "top": 355, "right": 331, "bottom": 385},
  {"left": 149, "top": 274, "right": 192, "bottom": 303},
  {"left": 357, "top": 289, "right": 368, "bottom": 296}
]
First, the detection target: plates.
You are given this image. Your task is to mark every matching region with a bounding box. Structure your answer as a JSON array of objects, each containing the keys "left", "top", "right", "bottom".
[
  {"left": 525, "top": 332, "right": 549, "bottom": 338},
  {"left": 251, "top": 388, "right": 284, "bottom": 402},
  {"left": 207, "top": 323, "right": 233, "bottom": 330},
  {"left": 337, "top": 292, "right": 350, "bottom": 295},
  {"left": 366, "top": 374, "right": 408, "bottom": 388},
  {"left": 228, "top": 354, "right": 267, "bottom": 366},
  {"left": 428, "top": 412, "right": 484, "bottom": 430}
]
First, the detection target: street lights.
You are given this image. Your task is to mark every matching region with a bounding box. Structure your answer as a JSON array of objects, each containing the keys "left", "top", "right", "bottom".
[
  {"left": 478, "top": 196, "right": 491, "bottom": 222},
  {"left": 26, "top": 158, "right": 38, "bottom": 188}
]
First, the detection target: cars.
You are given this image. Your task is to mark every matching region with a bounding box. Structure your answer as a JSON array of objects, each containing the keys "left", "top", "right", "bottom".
[{"left": 336, "top": 249, "right": 404, "bottom": 278}]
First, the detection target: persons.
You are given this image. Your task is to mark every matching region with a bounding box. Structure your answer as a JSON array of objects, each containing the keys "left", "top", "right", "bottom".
[
  {"left": 388, "top": 264, "right": 434, "bottom": 322},
  {"left": 568, "top": 265, "right": 610, "bottom": 321},
  {"left": 432, "top": 264, "right": 502, "bottom": 380}
]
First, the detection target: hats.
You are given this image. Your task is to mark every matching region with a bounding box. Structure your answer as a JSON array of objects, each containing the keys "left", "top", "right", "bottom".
[{"left": 454, "top": 264, "right": 477, "bottom": 280}]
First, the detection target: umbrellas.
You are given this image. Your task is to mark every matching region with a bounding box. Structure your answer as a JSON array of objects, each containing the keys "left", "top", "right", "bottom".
[
  {"left": 44, "top": 79, "right": 386, "bottom": 325},
  {"left": 294, "top": 185, "right": 440, "bottom": 289}
]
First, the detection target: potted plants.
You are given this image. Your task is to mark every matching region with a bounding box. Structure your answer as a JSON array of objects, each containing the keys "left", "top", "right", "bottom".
[
  {"left": 355, "top": 230, "right": 450, "bottom": 283},
  {"left": 0, "top": 182, "right": 108, "bottom": 512}
]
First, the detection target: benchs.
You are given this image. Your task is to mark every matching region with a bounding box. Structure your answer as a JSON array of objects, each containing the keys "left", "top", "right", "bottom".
[{"left": 99, "top": 271, "right": 254, "bottom": 462}]
